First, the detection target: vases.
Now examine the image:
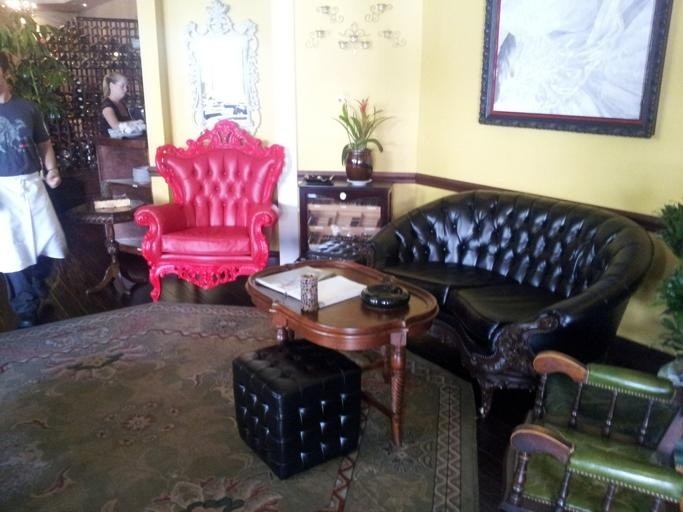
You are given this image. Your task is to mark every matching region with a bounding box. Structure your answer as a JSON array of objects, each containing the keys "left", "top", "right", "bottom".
[{"left": 345, "top": 149, "right": 373, "bottom": 187}]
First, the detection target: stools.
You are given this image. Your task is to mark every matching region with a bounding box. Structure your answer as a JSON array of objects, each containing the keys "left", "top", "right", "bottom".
[{"left": 233, "top": 338, "right": 362, "bottom": 479}]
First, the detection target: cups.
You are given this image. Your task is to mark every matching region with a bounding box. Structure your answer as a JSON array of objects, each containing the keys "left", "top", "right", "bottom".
[{"left": 299, "top": 272, "right": 318, "bottom": 313}]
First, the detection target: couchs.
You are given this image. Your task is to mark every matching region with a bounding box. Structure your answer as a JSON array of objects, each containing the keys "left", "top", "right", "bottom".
[{"left": 355, "top": 190, "right": 654, "bottom": 416}]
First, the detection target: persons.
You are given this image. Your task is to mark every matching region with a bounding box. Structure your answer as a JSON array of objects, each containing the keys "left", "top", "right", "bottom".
[
  {"left": 0, "top": 50, "right": 69, "bottom": 332},
  {"left": 100, "top": 71, "right": 133, "bottom": 136}
]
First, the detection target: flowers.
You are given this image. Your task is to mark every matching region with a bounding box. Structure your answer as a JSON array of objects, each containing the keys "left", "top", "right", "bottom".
[{"left": 334, "top": 97, "right": 393, "bottom": 165}]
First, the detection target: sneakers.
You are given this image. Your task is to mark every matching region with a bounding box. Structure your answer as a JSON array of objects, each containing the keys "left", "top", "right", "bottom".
[
  {"left": 17, "top": 311, "right": 40, "bottom": 328},
  {"left": 31, "top": 272, "right": 50, "bottom": 299}
]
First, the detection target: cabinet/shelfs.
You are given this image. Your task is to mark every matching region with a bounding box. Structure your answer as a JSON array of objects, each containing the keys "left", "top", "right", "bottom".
[{"left": 39, "top": 17, "right": 145, "bottom": 178}]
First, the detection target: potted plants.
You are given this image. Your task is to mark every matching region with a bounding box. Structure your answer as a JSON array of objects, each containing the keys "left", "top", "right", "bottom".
[{"left": 654, "top": 202, "right": 683, "bottom": 389}]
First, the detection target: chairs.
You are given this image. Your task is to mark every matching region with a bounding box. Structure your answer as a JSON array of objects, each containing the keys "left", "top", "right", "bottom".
[
  {"left": 498, "top": 351, "right": 683, "bottom": 512},
  {"left": 134, "top": 120, "right": 284, "bottom": 302}
]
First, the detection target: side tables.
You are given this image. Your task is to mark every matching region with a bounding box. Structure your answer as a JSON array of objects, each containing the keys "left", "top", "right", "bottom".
[
  {"left": 64, "top": 192, "right": 146, "bottom": 296},
  {"left": 300, "top": 181, "right": 391, "bottom": 261}
]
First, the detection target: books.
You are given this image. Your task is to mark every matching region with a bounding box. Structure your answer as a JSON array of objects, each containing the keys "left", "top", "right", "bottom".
[
  {"left": 256, "top": 264, "right": 335, "bottom": 295},
  {"left": 288, "top": 275, "right": 367, "bottom": 309}
]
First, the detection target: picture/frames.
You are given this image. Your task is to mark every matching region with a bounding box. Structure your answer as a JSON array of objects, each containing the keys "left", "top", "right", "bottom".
[{"left": 478, "top": 0, "right": 674, "bottom": 138}]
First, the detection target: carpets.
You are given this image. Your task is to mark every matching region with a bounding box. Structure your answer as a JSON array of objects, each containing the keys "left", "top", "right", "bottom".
[{"left": 0, "top": 302, "right": 480, "bottom": 512}]
